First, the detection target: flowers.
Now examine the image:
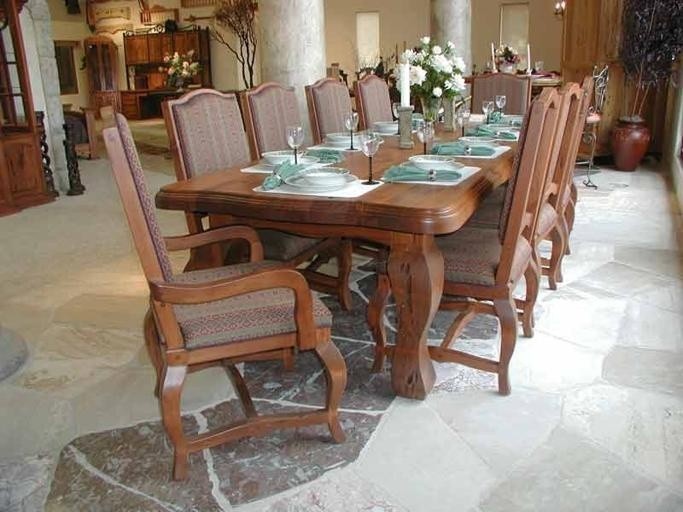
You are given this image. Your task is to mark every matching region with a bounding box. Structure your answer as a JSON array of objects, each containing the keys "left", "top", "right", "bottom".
[
  {"left": 157, "top": 50, "right": 202, "bottom": 78},
  {"left": 494, "top": 45, "right": 520, "bottom": 67},
  {"left": 391, "top": 35, "right": 466, "bottom": 100}
]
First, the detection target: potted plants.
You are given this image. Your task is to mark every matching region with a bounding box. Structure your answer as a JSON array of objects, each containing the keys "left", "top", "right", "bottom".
[{"left": 611, "top": 0, "right": 683, "bottom": 172}]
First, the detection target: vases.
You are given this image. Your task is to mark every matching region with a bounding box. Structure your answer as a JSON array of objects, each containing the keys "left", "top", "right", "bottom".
[{"left": 498, "top": 65, "right": 512, "bottom": 73}]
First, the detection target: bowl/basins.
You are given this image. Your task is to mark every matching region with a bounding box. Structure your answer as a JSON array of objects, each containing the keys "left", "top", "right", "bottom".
[
  {"left": 407, "top": 153, "right": 454, "bottom": 169},
  {"left": 326, "top": 132, "right": 358, "bottom": 141},
  {"left": 261, "top": 148, "right": 302, "bottom": 164},
  {"left": 374, "top": 121, "right": 398, "bottom": 131},
  {"left": 457, "top": 136, "right": 498, "bottom": 147},
  {"left": 299, "top": 166, "right": 349, "bottom": 185},
  {"left": 487, "top": 123, "right": 513, "bottom": 131}
]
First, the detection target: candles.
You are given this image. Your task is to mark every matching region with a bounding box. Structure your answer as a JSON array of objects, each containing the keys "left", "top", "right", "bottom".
[
  {"left": 399, "top": 60, "right": 411, "bottom": 107},
  {"left": 491, "top": 42, "right": 496, "bottom": 69},
  {"left": 527, "top": 43, "right": 532, "bottom": 76}
]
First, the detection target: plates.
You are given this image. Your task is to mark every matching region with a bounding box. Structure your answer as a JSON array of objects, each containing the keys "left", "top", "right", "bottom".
[
  {"left": 285, "top": 173, "right": 359, "bottom": 192},
  {"left": 322, "top": 137, "right": 353, "bottom": 148},
  {"left": 260, "top": 155, "right": 319, "bottom": 169}
]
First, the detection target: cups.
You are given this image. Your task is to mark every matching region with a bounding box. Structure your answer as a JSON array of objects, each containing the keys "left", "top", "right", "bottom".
[{"left": 534, "top": 60, "right": 544, "bottom": 73}]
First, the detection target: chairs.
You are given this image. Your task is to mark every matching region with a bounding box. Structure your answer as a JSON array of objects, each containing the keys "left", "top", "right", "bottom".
[
  {"left": 101, "top": 112, "right": 348, "bottom": 482},
  {"left": 575, "top": 64, "right": 609, "bottom": 188}
]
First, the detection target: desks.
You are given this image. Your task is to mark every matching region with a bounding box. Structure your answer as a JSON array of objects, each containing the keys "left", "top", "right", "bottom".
[{"left": 464, "top": 74, "right": 564, "bottom": 100}]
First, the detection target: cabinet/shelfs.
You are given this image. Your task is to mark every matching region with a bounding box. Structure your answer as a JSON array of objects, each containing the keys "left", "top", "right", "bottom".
[
  {"left": 83, "top": 33, "right": 119, "bottom": 120},
  {"left": 120, "top": 26, "right": 215, "bottom": 120}
]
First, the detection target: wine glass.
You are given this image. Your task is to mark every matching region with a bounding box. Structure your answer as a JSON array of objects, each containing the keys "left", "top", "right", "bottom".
[
  {"left": 455, "top": 108, "right": 471, "bottom": 136},
  {"left": 359, "top": 129, "right": 382, "bottom": 185},
  {"left": 285, "top": 123, "right": 305, "bottom": 166},
  {"left": 414, "top": 119, "right": 435, "bottom": 153},
  {"left": 495, "top": 94, "right": 507, "bottom": 119},
  {"left": 482, "top": 100, "right": 495, "bottom": 126},
  {"left": 392, "top": 103, "right": 402, "bottom": 120},
  {"left": 343, "top": 113, "right": 360, "bottom": 151}
]
